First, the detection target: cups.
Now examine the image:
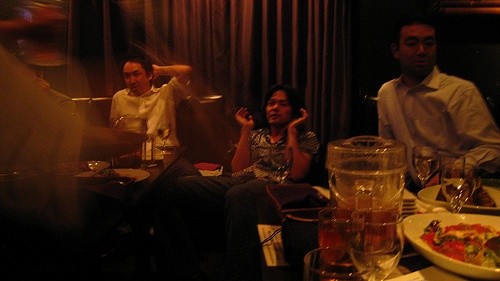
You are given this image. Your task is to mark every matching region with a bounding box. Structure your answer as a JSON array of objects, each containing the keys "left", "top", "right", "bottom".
[
  {"left": 151, "top": 140, "right": 165, "bottom": 164},
  {"left": 303, "top": 246, "right": 375, "bottom": 281},
  {"left": 348, "top": 208, "right": 405, "bottom": 281},
  {"left": 317, "top": 206, "right": 365, "bottom": 274}
]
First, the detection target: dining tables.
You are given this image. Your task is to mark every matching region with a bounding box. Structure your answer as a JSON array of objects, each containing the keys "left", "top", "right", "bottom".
[
  {"left": 0, "top": 138, "right": 190, "bottom": 221},
  {"left": 257, "top": 171, "right": 500, "bottom": 281}
]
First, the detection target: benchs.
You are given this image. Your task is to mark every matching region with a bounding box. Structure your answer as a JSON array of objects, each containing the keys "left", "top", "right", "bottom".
[{"left": 36, "top": 94, "right": 327, "bottom": 281}]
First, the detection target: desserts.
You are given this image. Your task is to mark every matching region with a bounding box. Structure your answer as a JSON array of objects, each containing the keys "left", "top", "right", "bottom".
[{"left": 436, "top": 179, "right": 497, "bottom": 208}]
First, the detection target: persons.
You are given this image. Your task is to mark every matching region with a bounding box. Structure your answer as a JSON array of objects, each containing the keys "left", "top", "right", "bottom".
[
  {"left": 376, "top": 13, "right": 500, "bottom": 193},
  {"left": 109, "top": 52, "right": 202, "bottom": 186},
  {"left": 153, "top": 84, "right": 320, "bottom": 281}
]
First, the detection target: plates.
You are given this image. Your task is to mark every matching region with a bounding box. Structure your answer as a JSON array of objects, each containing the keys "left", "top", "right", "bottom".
[
  {"left": 72, "top": 168, "right": 151, "bottom": 182},
  {"left": 401, "top": 212, "right": 500, "bottom": 280},
  {"left": 417, "top": 183, "right": 500, "bottom": 216},
  {"left": 87, "top": 161, "right": 110, "bottom": 169}
]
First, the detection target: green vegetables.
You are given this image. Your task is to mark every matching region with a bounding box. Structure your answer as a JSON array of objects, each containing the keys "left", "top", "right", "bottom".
[{"left": 436, "top": 225, "right": 495, "bottom": 260}]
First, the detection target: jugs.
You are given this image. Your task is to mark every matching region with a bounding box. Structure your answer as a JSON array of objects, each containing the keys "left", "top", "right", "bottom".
[
  {"left": 110, "top": 115, "right": 146, "bottom": 169},
  {"left": 325, "top": 136, "right": 407, "bottom": 254}
]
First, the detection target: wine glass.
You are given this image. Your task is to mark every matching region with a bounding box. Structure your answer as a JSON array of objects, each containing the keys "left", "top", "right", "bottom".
[
  {"left": 412, "top": 145, "right": 441, "bottom": 189},
  {"left": 441, "top": 162, "right": 477, "bottom": 212}
]
